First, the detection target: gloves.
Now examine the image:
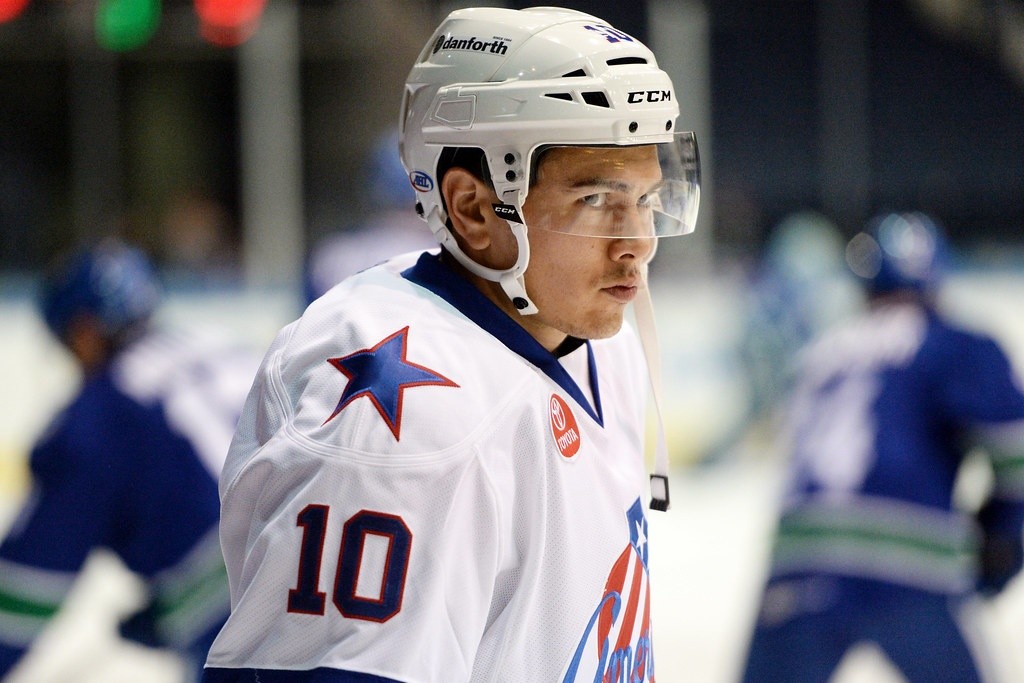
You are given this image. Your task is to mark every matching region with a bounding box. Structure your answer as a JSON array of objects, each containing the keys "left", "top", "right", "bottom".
[{"left": 972, "top": 495, "right": 1024, "bottom": 597}]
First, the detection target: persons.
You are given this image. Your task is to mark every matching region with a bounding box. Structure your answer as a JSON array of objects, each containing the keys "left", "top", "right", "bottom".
[
  {"left": 302, "top": 138, "right": 439, "bottom": 312},
  {"left": 203, "top": 7, "right": 701, "bottom": 683},
  {"left": 0, "top": 243, "right": 250, "bottom": 683},
  {"left": 740, "top": 210, "right": 1023, "bottom": 683}
]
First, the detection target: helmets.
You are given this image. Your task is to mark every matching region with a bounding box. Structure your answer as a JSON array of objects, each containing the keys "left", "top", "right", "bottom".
[
  {"left": 36, "top": 243, "right": 159, "bottom": 342},
  {"left": 849, "top": 212, "right": 946, "bottom": 292},
  {"left": 397, "top": 6, "right": 702, "bottom": 317},
  {"left": 375, "top": 128, "right": 420, "bottom": 207}
]
